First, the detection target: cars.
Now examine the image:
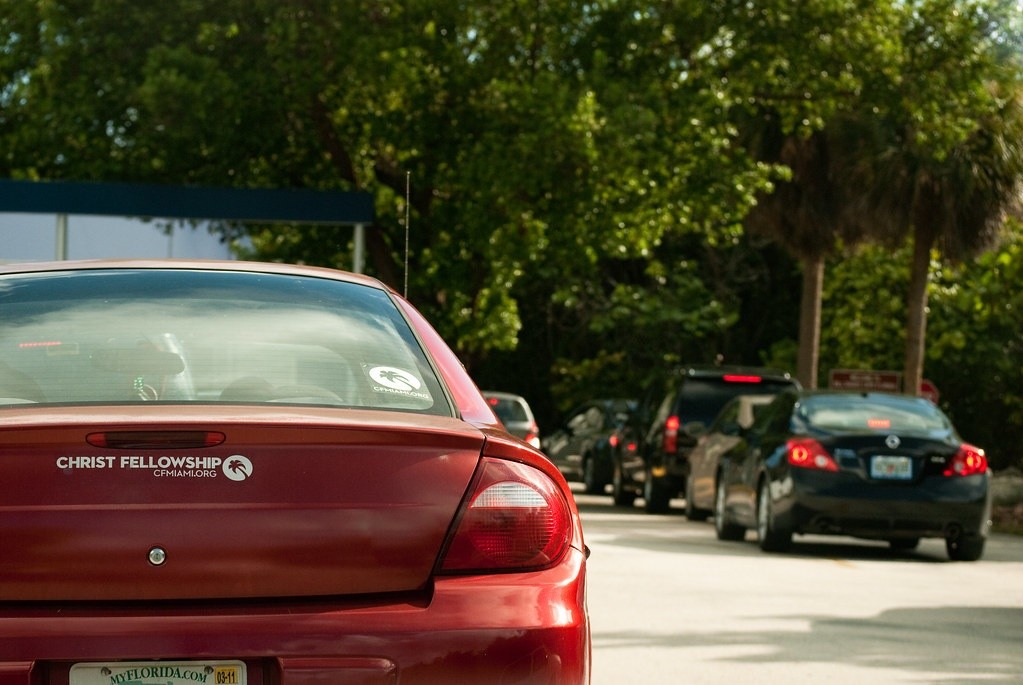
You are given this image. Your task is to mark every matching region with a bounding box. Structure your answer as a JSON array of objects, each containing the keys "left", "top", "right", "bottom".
[
  {"left": 0, "top": 260, "right": 591, "bottom": 685},
  {"left": 712, "top": 388, "right": 994, "bottom": 563},
  {"left": 479, "top": 389, "right": 540, "bottom": 450},
  {"left": 541, "top": 399, "right": 641, "bottom": 494},
  {"left": 683, "top": 391, "right": 776, "bottom": 521}
]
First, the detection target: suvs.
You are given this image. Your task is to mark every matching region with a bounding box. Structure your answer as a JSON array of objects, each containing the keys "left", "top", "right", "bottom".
[{"left": 607, "top": 366, "right": 805, "bottom": 514}]
